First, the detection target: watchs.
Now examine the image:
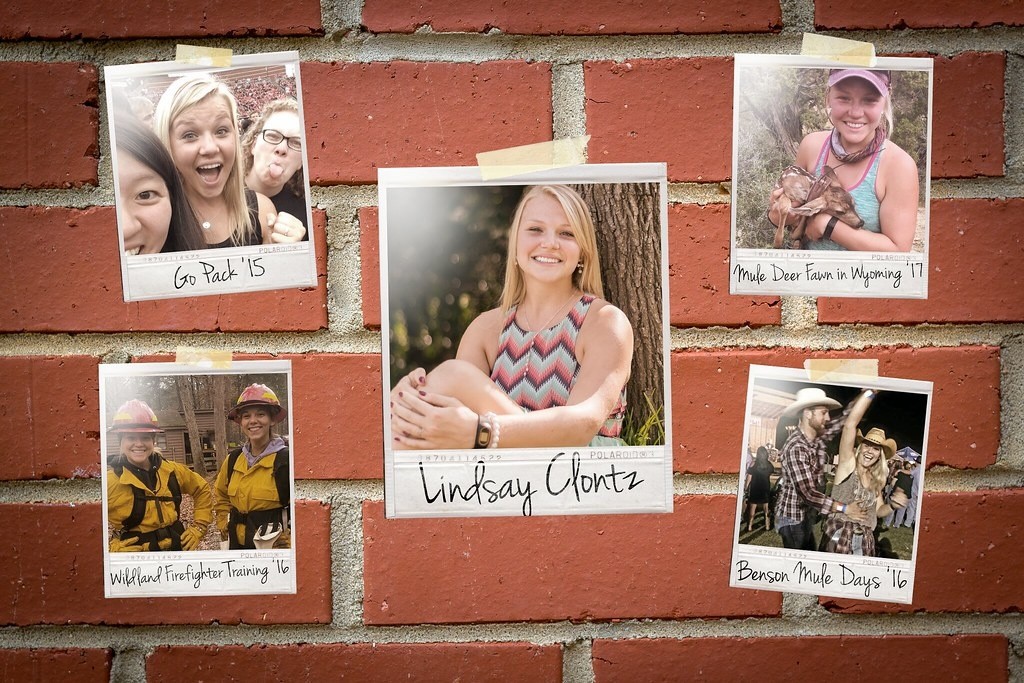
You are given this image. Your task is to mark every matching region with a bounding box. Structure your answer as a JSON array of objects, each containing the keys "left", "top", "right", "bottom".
[
  {"left": 863, "top": 389, "right": 876, "bottom": 399},
  {"left": 836, "top": 500, "right": 843, "bottom": 514},
  {"left": 471, "top": 414, "right": 492, "bottom": 449}
]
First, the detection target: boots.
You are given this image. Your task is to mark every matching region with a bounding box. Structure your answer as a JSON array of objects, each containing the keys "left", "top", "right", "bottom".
[
  {"left": 749, "top": 514, "right": 755, "bottom": 531},
  {"left": 764, "top": 513, "right": 770, "bottom": 531}
]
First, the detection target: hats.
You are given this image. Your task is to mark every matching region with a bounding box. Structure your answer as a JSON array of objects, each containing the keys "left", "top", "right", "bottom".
[
  {"left": 913, "top": 456, "right": 921, "bottom": 464},
  {"left": 827, "top": 69, "right": 891, "bottom": 98},
  {"left": 856, "top": 428, "right": 897, "bottom": 459},
  {"left": 784, "top": 388, "right": 843, "bottom": 417}
]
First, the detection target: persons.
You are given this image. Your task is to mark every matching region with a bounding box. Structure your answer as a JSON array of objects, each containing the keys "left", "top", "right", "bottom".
[
  {"left": 113, "top": 108, "right": 209, "bottom": 257},
  {"left": 743, "top": 380, "right": 923, "bottom": 557},
  {"left": 390, "top": 184, "right": 634, "bottom": 451},
  {"left": 211, "top": 382, "right": 292, "bottom": 550},
  {"left": 151, "top": 72, "right": 280, "bottom": 252},
  {"left": 240, "top": 98, "right": 309, "bottom": 243},
  {"left": 105, "top": 398, "right": 215, "bottom": 555},
  {"left": 768, "top": 68, "right": 920, "bottom": 252}
]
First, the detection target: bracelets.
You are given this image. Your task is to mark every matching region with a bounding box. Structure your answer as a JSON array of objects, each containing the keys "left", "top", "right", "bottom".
[
  {"left": 824, "top": 216, "right": 839, "bottom": 242},
  {"left": 888, "top": 503, "right": 896, "bottom": 511},
  {"left": 842, "top": 502, "right": 848, "bottom": 514},
  {"left": 485, "top": 412, "right": 501, "bottom": 448}
]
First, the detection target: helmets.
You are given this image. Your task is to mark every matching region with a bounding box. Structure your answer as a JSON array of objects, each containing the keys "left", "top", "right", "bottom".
[
  {"left": 109, "top": 399, "right": 164, "bottom": 433},
  {"left": 228, "top": 383, "right": 287, "bottom": 424}
]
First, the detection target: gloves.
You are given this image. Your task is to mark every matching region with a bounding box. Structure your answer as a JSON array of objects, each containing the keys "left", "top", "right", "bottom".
[
  {"left": 272, "top": 528, "right": 291, "bottom": 549},
  {"left": 109, "top": 536, "right": 143, "bottom": 552},
  {"left": 180, "top": 522, "right": 208, "bottom": 551},
  {"left": 220, "top": 523, "right": 228, "bottom": 542}
]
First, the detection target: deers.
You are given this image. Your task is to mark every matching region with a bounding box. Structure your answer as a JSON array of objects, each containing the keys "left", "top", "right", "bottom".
[{"left": 770, "top": 163, "right": 866, "bottom": 250}]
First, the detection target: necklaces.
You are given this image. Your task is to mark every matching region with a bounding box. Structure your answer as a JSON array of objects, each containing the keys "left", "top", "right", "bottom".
[
  {"left": 190, "top": 194, "right": 225, "bottom": 231},
  {"left": 521, "top": 285, "right": 580, "bottom": 413}
]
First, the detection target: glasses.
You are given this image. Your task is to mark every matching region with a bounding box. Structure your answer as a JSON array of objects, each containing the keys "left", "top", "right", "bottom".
[{"left": 259, "top": 129, "right": 301, "bottom": 152}]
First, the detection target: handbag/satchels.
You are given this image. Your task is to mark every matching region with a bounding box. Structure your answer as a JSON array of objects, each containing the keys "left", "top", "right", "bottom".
[{"left": 253, "top": 508, "right": 283, "bottom": 549}]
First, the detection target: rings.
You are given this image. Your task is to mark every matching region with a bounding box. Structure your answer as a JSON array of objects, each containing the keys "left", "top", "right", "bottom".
[{"left": 284, "top": 227, "right": 291, "bottom": 237}]
